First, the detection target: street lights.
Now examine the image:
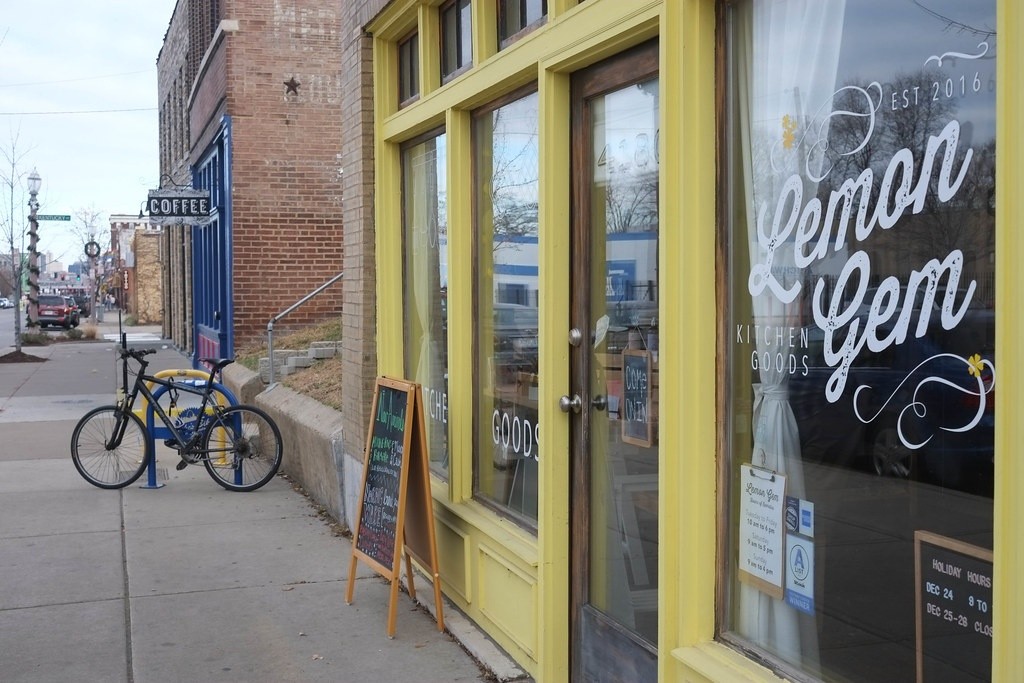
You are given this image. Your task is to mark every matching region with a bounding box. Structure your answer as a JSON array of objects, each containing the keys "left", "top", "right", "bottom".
[
  {"left": 27, "top": 167, "right": 41, "bottom": 331},
  {"left": 86, "top": 223, "right": 97, "bottom": 323}
]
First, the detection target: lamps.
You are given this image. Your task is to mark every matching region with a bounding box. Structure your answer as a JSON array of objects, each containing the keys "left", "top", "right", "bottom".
[{"left": 137, "top": 201, "right": 148, "bottom": 218}]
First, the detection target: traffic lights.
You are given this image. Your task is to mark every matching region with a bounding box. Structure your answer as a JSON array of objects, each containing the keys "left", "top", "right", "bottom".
[{"left": 61, "top": 275, "right": 64, "bottom": 281}]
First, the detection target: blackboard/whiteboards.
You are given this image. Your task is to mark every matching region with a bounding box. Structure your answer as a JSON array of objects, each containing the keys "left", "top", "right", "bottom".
[
  {"left": 622, "top": 352, "right": 651, "bottom": 445},
  {"left": 349, "top": 374, "right": 413, "bottom": 573},
  {"left": 913, "top": 528, "right": 996, "bottom": 683}
]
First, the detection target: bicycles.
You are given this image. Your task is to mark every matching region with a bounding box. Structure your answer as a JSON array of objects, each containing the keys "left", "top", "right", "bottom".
[{"left": 70, "top": 348, "right": 284, "bottom": 492}]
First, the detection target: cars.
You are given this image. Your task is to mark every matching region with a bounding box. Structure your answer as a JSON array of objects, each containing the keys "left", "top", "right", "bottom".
[
  {"left": 83, "top": 290, "right": 91, "bottom": 301},
  {"left": 492, "top": 303, "right": 537, "bottom": 370},
  {"left": 28, "top": 294, "right": 76, "bottom": 330},
  {"left": 63, "top": 296, "right": 81, "bottom": 327},
  {"left": 751, "top": 312, "right": 995, "bottom": 483},
  {"left": 845, "top": 285, "right": 986, "bottom": 314},
  {"left": 0, "top": 298, "right": 9, "bottom": 308}
]
